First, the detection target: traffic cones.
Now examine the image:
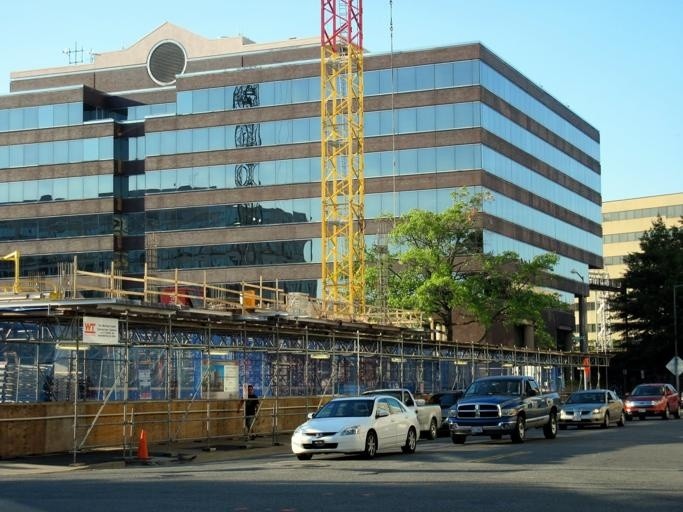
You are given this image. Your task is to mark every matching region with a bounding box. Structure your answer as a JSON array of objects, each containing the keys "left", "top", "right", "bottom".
[{"left": 137, "top": 429, "right": 148, "bottom": 459}]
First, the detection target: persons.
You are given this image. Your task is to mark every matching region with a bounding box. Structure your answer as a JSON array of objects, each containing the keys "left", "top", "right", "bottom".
[{"left": 237, "top": 383, "right": 262, "bottom": 441}]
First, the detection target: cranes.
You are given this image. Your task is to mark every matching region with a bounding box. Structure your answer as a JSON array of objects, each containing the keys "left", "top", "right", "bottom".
[{"left": 320, "top": 2, "right": 366, "bottom": 320}]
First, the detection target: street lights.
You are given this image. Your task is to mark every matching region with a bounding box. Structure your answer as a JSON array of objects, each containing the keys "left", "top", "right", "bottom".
[{"left": 571, "top": 268, "right": 585, "bottom": 352}]
